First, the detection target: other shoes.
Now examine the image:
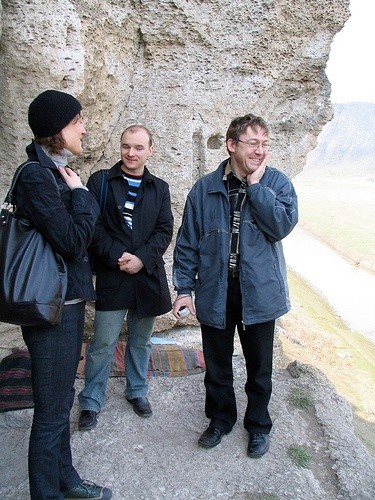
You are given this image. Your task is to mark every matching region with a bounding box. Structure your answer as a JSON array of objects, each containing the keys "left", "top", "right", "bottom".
[
  {"left": 198, "top": 427, "right": 225, "bottom": 448},
  {"left": 246, "top": 433, "right": 269, "bottom": 459},
  {"left": 126, "top": 397, "right": 153, "bottom": 419},
  {"left": 78, "top": 410, "right": 97, "bottom": 431}
]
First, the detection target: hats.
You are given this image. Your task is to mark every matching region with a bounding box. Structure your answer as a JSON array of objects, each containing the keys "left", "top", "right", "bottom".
[{"left": 28, "top": 90, "right": 82, "bottom": 139}]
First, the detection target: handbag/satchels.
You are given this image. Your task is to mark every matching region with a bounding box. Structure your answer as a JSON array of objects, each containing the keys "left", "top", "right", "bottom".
[{"left": 0, "top": 162, "right": 68, "bottom": 326}]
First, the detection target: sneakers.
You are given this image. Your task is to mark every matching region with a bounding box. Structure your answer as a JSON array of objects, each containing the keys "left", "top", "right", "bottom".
[{"left": 62, "top": 479, "right": 112, "bottom": 500}]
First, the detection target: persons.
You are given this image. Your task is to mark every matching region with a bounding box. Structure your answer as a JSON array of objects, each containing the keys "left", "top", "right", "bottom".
[
  {"left": 5, "top": 90, "right": 112, "bottom": 500},
  {"left": 172, "top": 113, "right": 298, "bottom": 458},
  {"left": 77, "top": 126, "right": 174, "bottom": 429}
]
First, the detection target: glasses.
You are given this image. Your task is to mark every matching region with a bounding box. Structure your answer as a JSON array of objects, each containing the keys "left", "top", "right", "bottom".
[{"left": 234, "top": 138, "right": 271, "bottom": 150}]
